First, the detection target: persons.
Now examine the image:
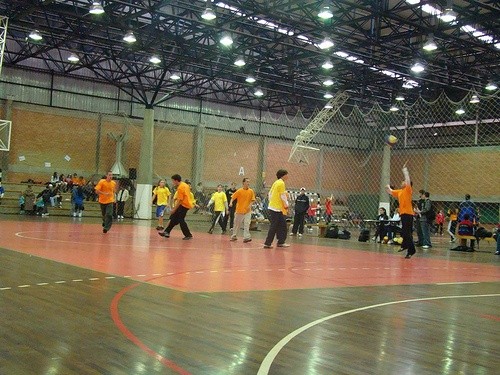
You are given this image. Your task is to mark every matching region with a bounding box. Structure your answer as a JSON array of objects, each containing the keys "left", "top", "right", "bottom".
[
  {"left": 94, "top": 171, "right": 117, "bottom": 234},
  {"left": 447, "top": 203, "right": 458, "bottom": 243},
  {"left": 372, "top": 189, "right": 433, "bottom": 248},
  {"left": 458, "top": 193, "right": 500, "bottom": 255},
  {"left": 385, "top": 159, "right": 416, "bottom": 258},
  {"left": 335, "top": 197, "right": 344, "bottom": 206},
  {"left": 436, "top": 210, "right": 444, "bottom": 236},
  {"left": 158, "top": 174, "right": 195, "bottom": 240},
  {"left": 152, "top": 180, "right": 340, "bottom": 224},
  {"left": 263, "top": 169, "right": 290, "bottom": 248},
  {"left": 224, "top": 182, "right": 237, "bottom": 230},
  {"left": 207, "top": 184, "right": 229, "bottom": 234},
  {"left": 343, "top": 210, "right": 366, "bottom": 227},
  {"left": 288, "top": 187, "right": 309, "bottom": 236},
  {"left": 153, "top": 179, "right": 171, "bottom": 230},
  {"left": 19, "top": 172, "right": 131, "bottom": 219},
  {"left": 229, "top": 177, "right": 255, "bottom": 243},
  {"left": 325, "top": 196, "right": 333, "bottom": 224}
]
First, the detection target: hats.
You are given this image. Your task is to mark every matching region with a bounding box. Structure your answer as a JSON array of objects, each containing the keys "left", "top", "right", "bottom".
[{"left": 300, "top": 187, "right": 305, "bottom": 191}]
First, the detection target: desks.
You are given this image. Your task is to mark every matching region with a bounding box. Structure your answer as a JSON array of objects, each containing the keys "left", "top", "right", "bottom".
[{"left": 363, "top": 219, "right": 401, "bottom": 245}]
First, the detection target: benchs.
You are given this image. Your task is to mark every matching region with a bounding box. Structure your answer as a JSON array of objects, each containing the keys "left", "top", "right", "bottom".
[
  {"left": 249, "top": 218, "right": 327, "bottom": 238},
  {"left": 455, "top": 234, "right": 497, "bottom": 253}
]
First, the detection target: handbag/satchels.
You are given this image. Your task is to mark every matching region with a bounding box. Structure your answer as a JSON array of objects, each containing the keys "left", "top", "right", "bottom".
[{"left": 424, "top": 199, "right": 435, "bottom": 221}]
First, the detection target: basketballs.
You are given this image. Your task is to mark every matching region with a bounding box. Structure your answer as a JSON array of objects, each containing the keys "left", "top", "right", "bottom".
[{"left": 384, "top": 135, "right": 398, "bottom": 145}]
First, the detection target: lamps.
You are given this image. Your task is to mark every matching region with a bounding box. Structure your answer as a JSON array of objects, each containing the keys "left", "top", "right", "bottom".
[
  {"left": 201, "top": 0, "right": 217, "bottom": 20},
  {"left": 123, "top": 24, "right": 137, "bottom": 42},
  {"left": 220, "top": 30, "right": 233, "bottom": 46},
  {"left": 234, "top": 51, "right": 263, "bottom": 96},
  {"left": 390, "top": 0, "right": 497, "bottom": 115},
  {"left": 149, "top": 52, "right": 161, "bottom": 64},
  {"left": 29, "top": 27, "right": 43, "bottom": 41},
  {"left": 170, "top": 72, "right": 180, "bottom": 80},
  {"left": 317, "top": 0, "right": 334, "bottom": 108},
  {"left": 68, "top": 51, "right": 80, "bottom": 62},
  {"left": 89, "top": 0, "right": 105, "bottom": 14}
]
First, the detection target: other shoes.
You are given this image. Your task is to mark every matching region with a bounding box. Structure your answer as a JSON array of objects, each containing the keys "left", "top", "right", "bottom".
[
  {"left": 230, "top": 235, "right": 238, "bottom": 241},
  {"left": 103, "top": 229, "right": 107, "bottom": 233},
  {"left": 121, "top": 216, "right": 124, "bottom": 219},
  {"left": 78, "top": 213, "right": 82, "bottom": 218},
  {"left": 158, "top": 232, "right": 169, "bottom": 238},
  {"left": 118, "top": 215, "right": 120, "bottom": 219},
  {"left": 289, "top": 233, "right": 296, "bottom": 236},
  {"left": 372, "top": 236, "right": 376, "bottom": 241},
  {"left": 277, "top": 244, "right": 290, "bottom": 247},
  {"left": 405, "top": 250, "right": 416, "bottom": 260},
  {"left": 73, "top": 213, "right": 77, "bottom": 217},
  {"left": 299, "top": 234, "right": 303, "bottom": 236},
  {"left": 182, "top": 234, "right": 192, "bottom": 240},
  {"left": 156, "top": 225, "right": 165, "bottom": 231},
  {"left": 398, "top": 248, "right": 407, "bottom": 252},
  {"left": 449, "top": 238, "right": 456, "bottom": 243},
  {"left": 422, "top": 246, "right": 429, "bottom": 249},
  {"left": 264, "top": 245, "right": 273, "bottom": 248},
  {"left": 243, "top": 238, "right": 252, "bottom": 243},
  {"left": 102, "top": 222, "right": 105, "bottom": 227}
]
